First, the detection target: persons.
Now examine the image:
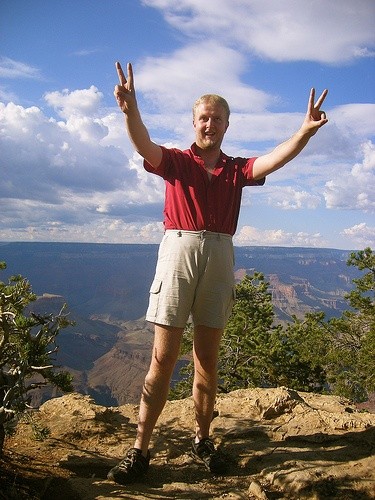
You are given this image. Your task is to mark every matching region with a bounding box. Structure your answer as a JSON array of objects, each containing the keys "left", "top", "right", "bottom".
[{"left": 107, "top": 61, "right": 329, "bottom": 485}]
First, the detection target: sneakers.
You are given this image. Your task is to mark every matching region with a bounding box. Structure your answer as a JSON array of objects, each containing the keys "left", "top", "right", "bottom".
[
  {"left": 192, "top": 435, "right": 227, "bottom": 475},
  {"left": 107, "top": 447, "right": 151, "bottom": 484}
]
